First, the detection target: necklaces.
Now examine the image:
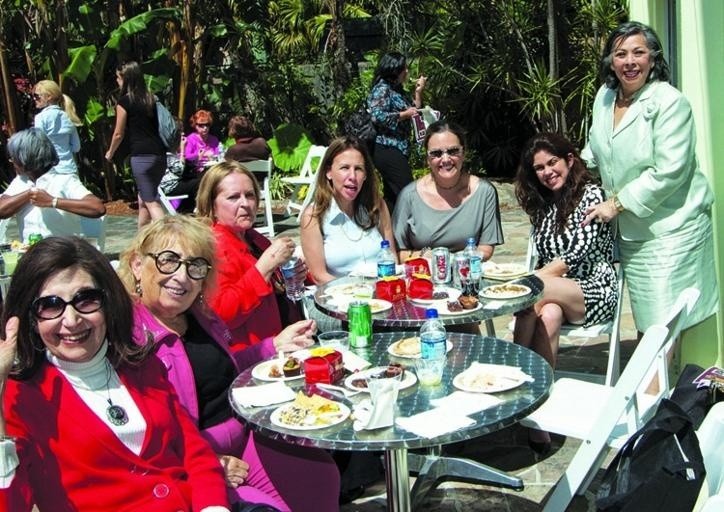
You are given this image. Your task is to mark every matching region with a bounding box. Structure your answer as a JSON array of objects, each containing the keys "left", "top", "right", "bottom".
[
  {"left": 63, "top": 362, "right": 132, "bottom": 427},
  {"left": 617, "top": 88, "right": 643, "bottom": 104}
]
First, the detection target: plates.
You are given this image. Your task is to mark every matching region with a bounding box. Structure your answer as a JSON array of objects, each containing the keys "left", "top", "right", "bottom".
[
  {"left": 270, "top": 398, "right": 352, "bottom": 432},
  {"left": 407, "top": 286, "right": 531, "bottom": 315},
  {"left": 346, "top": 366, "right": 416, "bottom": 392},
  {"left": 14, "top": 246, "right": 28, "bottom": 252},
  {"left": 252, "top": 358, "right": 306, "bottom": 381},
  {"left": 455, "top": 363, "right": 526, "bottom": 392},
  {"left": 481, "top": 263, "right": 527, "bottom": 277},
  {"left": 385, "top": 337, "right": 453, "bottom": 358},
  {"left": 349, "top": 262, "right": 404, "bottom": 277},
  {"left": 326, "top": 285, "right": 393, "bottom": 316}
]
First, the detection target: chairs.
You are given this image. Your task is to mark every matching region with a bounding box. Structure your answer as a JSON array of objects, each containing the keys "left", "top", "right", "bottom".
[
  {"left": 156, "top": 183, "right": 189, "bottom": 218},
  {"left": 77, "top": 211, "right": 110, "bottom": 252},
  {"left": 231, "top": 155, "right": 277, "bottom": 239},
  {"left": 506, "top": 233, "right": 626, "bottom": 387},
  {"left": 518, "top": 284, "right": 702, "bottom": 512},
  {"left": 289, "top": 245, "right": 318, "bottom": 324},
  {"left": 279, "top": 144, "right": 331, "bottom": 222}
]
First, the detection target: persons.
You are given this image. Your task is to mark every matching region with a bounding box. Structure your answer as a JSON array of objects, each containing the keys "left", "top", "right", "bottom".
[
  {"left": 499, "top": 134, "right": 619, "bottom": 455},
  {"left": 393, "top": 120, "right": 504, "bottom": 336},
  {"left": 0, "top": 236, "right": 244, "bottom": 511},
  {"left": 190, "top": 160, "right": 320, "bottom": 355},
  {"left": 299, "top": 136, "right": 400, "bottom": 292},
  {"left": 115, "top": 215, "right": 342, "bottom": 512},
  {"left": 576, "top": 21, "right": 718, "bottom": 403}
]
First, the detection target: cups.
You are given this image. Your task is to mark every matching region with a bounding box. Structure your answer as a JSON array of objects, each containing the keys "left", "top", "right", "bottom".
[
  {"left": 414, "top": 355, "right": 446, "bottom": 386},
  {"left": 317, "top": 331, "right": 352, "bottom": 358},
  {"left": 1, "top": 250, "right": 19, "bottom": 264},
  {"left": 365, "top": 367, "right": 403, "bottom": 426}
]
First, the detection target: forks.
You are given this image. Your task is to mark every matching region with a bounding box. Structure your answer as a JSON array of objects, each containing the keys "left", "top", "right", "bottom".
[{"left": 317, "top": 382, "right": 363, "bottom": 397}]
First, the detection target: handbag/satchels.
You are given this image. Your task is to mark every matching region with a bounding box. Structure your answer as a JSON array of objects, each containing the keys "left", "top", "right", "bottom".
[{"left": 595, "top": 399, "right": 707, "bottom": 512}]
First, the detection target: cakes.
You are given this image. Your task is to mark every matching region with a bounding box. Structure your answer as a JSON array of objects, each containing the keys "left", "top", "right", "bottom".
[{"left": 282, "top": 355, "right": 301, "bottom": 378}]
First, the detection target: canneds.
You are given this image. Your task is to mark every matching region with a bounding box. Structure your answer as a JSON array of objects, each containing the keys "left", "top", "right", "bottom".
[
  {"left": 432, "top": 246, "right": 451, "bottom": 284},
  {"left": 348, "top": 302, "right": 373, "bottom": 348},
  {"left": 452, "top": 250, "right": 470, "bottom": 291},
  {"left": 28, "top": 233, "right": 42, "bottom": 247}
]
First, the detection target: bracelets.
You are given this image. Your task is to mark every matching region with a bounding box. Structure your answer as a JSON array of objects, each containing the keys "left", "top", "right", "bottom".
[{"left": 613, "top": 194, "right": 628, "bottom": 216}]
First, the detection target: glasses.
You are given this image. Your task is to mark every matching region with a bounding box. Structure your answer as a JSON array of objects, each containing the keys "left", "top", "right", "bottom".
[
  {"left": 144, "top": 250, "right": 213, "bottom": 282},
  {"left": 30, "top": 287, "right": 107, "bottom": 320},
  {"left": 426, "top": 146, "right": 467, "bottom": 159}
]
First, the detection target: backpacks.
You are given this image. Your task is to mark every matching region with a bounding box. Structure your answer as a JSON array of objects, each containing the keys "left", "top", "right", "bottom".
[
  {"left": 345, "top": 100, "right": 378, "bottom": 148},
  {"left": 153, "top": 94, "right": 182, "bottom": 151}
]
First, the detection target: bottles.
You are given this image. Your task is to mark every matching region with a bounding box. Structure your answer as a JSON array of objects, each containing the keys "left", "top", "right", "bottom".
[
  {"left": 376, "top": 240, "right": 397, "bottom": 280},
  {"left": 282, "top": 244, "right": 305, "bottom": 301},
  {"left": 420, "top": 308, "right": 449, "bottom": 369},
  {"left": 218, "top": 142, "right": 225, "bottom": 161},
  {"left": 464, "top": 238, "right": 481, "bottom": 295}
]
